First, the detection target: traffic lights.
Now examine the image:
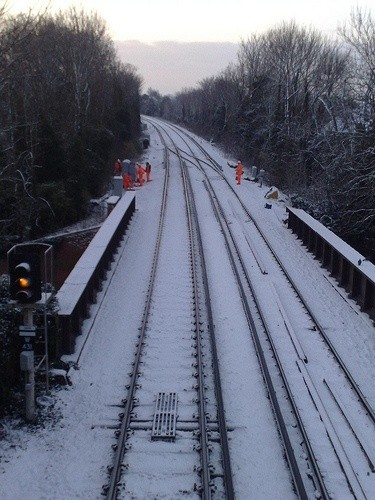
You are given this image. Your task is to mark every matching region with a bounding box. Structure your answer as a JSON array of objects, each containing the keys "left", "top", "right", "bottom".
[{"left": 12, "top": 254, "right": 42, "bottom": 303}]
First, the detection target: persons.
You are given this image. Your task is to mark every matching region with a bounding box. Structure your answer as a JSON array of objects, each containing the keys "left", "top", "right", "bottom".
[
  {"left": 235, "top": 161, "right": 243, "bottom": 185},
  {"left": 110, "top": 159, "right": 151, "bottom": 190}
]
